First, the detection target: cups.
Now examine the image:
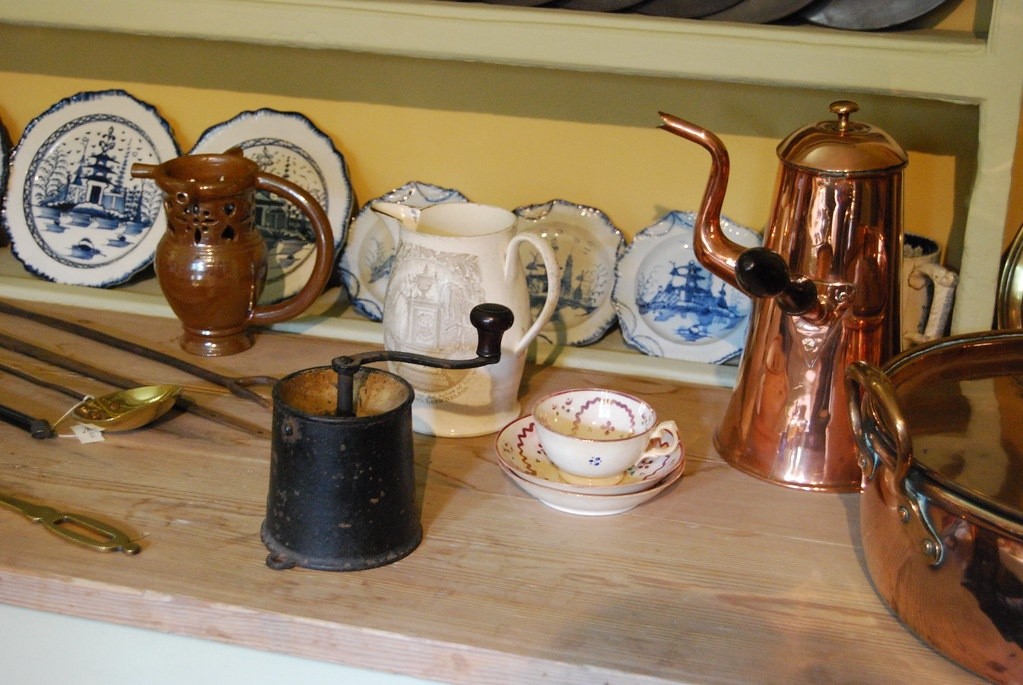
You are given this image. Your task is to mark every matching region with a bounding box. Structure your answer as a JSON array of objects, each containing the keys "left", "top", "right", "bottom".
[
  {"left": 900, "top": 233, "right": 956, "bottom": 355},
  {"left": 532, "top": 388, "right": 680, "bottom": 485}
]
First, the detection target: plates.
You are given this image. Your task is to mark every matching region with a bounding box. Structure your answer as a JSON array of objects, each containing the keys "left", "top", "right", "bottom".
[
  {"left": 513, "top": 199, "right": 626, "bottom": 346},
  {"left": 496, "top": 414, "right": 685, "bottom": 516},
  {"left": 188, "top": 108, "right": 353, "bottom": 305},
  {"left": 341, "top": 180, "right": 469, "bottom": 323},
  {"left": 612, "top": 210, "right": 763, "bottom": 364},
  {"left": 3, "top": 89, "right": 179, "bottom": 289}
]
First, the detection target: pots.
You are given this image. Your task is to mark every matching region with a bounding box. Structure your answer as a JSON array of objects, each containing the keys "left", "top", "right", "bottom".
[{"left": 845, "top": 330, "right": 1023, "bottom": 685}]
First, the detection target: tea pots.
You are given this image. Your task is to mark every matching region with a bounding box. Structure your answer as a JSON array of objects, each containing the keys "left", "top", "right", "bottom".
[{"left": 655, "top": 100, "right": 910, "bottom": 494}]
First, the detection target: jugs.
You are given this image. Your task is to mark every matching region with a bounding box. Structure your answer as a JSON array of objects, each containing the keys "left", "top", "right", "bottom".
[{"left": 369, "top": 202, "right": 561, "bottom": 438}]
